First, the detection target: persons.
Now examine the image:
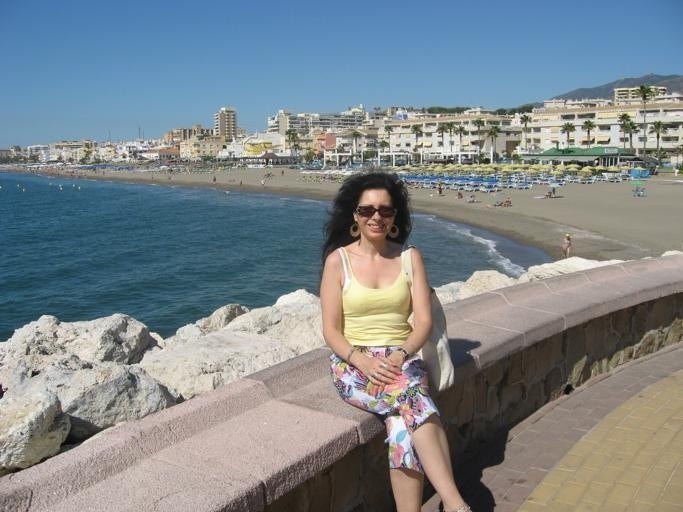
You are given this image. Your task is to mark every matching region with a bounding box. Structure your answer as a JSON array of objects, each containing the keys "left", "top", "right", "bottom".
[
  {"left": 434, "top": 182, "right": 561, "bottom": 209},
  {"left": 316, "top": 168, "right": 472, "bottom": 512},
  {"left": 561, "top": 234, "right": 571, "bottom": 258}
]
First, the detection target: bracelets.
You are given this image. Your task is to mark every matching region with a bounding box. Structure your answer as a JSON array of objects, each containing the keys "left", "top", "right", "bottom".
[
  {"left": 394, "top": 347, "right": 409, "bottom": 360},
  {"left": 346, "top": 347, "right": 355, "bottom": 365}
]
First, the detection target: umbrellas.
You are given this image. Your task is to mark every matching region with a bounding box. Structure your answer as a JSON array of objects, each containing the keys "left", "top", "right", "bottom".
[
  {"left": 628, "top": 181, "right": 643, "bottom": 186},
  {"left": 387, "top": 162, "right": 644, "bottom": 181}
]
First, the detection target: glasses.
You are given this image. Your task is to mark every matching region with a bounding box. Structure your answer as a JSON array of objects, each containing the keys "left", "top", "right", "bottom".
[{"left": 356, "top": 205, "right": 396, "bottom": 218}]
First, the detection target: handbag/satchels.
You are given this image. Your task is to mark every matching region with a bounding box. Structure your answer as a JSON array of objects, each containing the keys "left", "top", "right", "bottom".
[{"left": 402, "top": 243, "right": 455, "bottom": 392}]
